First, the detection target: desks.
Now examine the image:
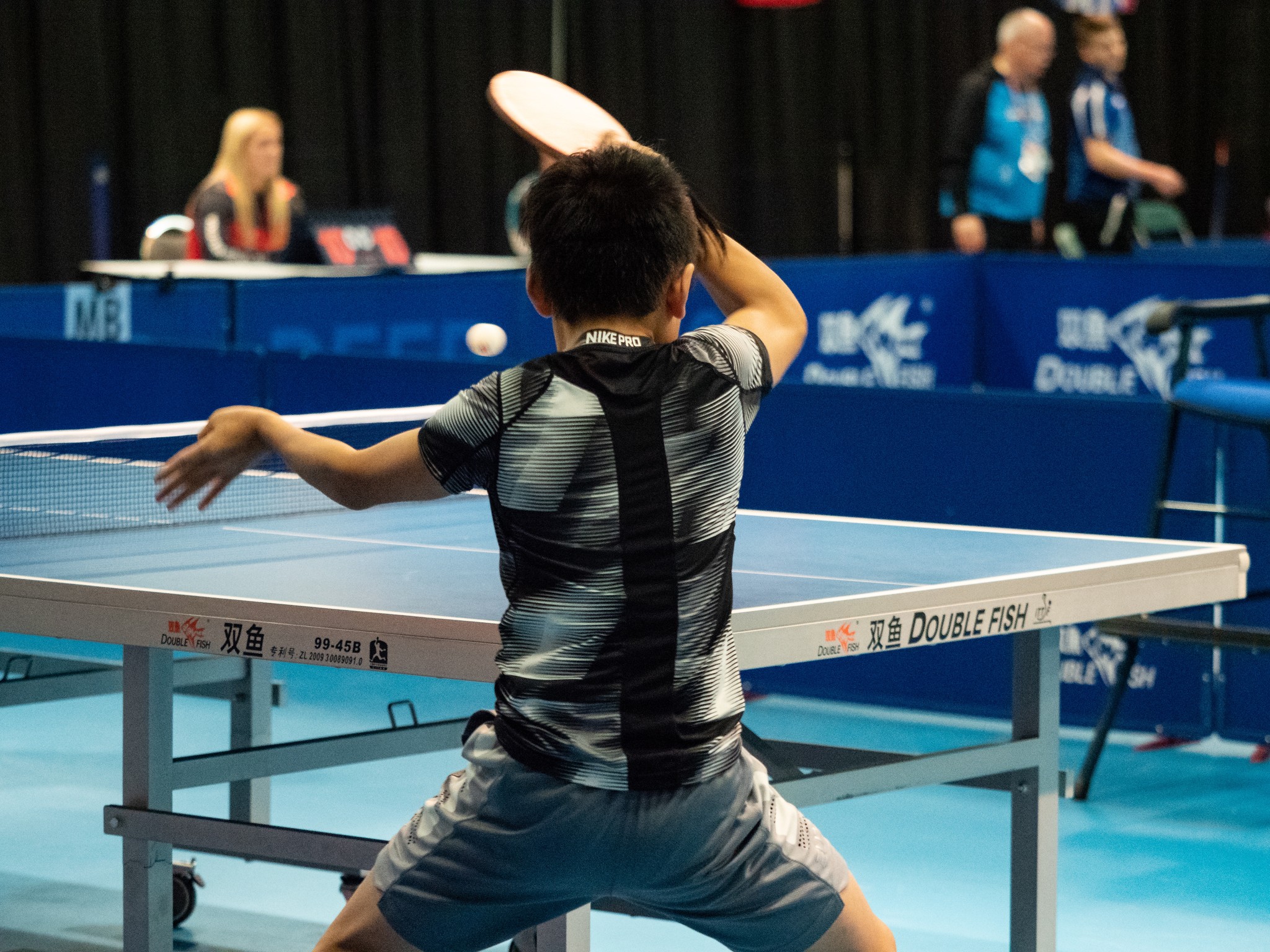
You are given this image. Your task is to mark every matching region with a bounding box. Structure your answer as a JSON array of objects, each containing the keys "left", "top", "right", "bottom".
[
  {"left": 0, "top": 399, "right": 1248, "bottom": 952},
  {"left": 80, "top": 256, "right": 526, "bottom": 278}
]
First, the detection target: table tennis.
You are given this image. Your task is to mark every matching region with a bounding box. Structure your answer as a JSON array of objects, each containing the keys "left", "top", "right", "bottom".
[{"left": 465, "top": 323, "right": 508, "bottom": 357}]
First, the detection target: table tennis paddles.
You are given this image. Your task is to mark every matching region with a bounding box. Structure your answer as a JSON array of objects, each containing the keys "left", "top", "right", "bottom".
[{"left": 486, "top": 70, "right": 634, "bottom": 162}]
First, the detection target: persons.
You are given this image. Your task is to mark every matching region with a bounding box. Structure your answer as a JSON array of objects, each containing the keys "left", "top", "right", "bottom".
[
  {"left": 182, "top": 106, "right": 322, "bottom": 262},
  {"left": 154, "top": 136, "right": 897, "bottom": 952},
  {"left": 937, "top": 6, "right": 1066, "bottom": 259},
  {"left": 1061, "top": 11, "right": 1183, "bottom": 259}
]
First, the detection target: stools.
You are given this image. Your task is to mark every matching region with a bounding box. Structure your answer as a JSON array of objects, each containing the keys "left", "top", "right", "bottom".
[{"left": 1070, "top": 293, "right": 1270, "bottom": 798}]
